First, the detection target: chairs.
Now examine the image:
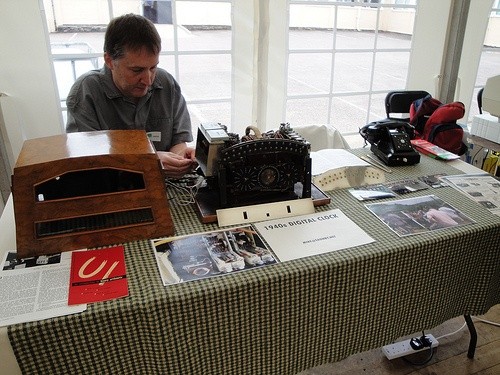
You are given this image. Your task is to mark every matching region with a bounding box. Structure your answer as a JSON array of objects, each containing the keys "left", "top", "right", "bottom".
[{"left": 294, "top": 125, "right": 349, "bottom": 162}]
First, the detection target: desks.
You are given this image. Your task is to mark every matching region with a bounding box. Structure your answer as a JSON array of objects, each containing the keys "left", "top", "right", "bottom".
[{"left": 1, "top": 139, "right": 500, "bottom": 374}]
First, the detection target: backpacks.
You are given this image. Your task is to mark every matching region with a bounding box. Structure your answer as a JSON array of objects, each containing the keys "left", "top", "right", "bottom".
[{"left": 410, "top": 95, "right": 466, "bottom": 154}]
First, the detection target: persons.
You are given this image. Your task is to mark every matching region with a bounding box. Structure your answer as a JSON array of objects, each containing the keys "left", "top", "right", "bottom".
[{"left": 66, "top": 12, "right": 198, "bottom": 180}]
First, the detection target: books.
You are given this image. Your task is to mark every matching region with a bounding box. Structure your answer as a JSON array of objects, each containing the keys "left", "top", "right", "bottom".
[{"left": 68, "top": 246, "right": 128, "bottom": 306}]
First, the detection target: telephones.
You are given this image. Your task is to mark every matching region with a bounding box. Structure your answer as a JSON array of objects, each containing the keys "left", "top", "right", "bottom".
[{"left": 360, "top": 119, "right": 422, "bottom": 168}]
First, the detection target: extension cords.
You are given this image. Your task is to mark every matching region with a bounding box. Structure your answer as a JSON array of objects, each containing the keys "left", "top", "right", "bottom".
[{"left": 380, "top": 333, "right": 440, "bottom": 362}]
